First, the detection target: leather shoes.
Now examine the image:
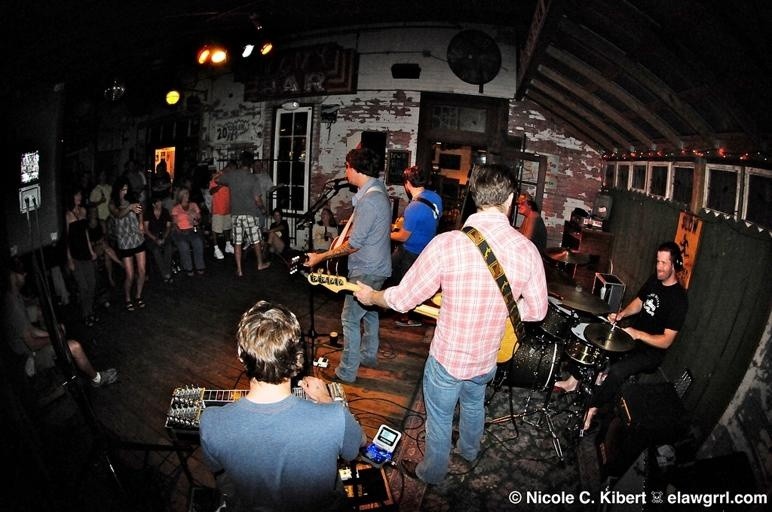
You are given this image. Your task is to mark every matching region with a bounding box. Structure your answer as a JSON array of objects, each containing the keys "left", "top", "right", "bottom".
[
  {"left": 320, "top": 368, "right": 344, "bottom": 382},
  {"left": 400, "top": 458, "right": 419, "bottom": 479}
]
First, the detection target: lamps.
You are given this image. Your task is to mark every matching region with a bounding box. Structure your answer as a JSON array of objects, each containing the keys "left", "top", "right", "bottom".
[{"left": 241, "top": 10, "right": 291, "bottom": 63}]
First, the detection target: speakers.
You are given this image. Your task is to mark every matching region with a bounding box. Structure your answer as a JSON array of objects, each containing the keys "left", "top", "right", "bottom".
[
  {"left": 591, "top": 272, "right": 625, "bottom": 313},
  {"left": 595, "top": 383, "right": 686, "bottom": 484},
  {"left": 391, "top": 63, "right": 420, "bottom": 79},
  {"left": 360, "top": 131, "right": 386, "bottom": 172},
  {"left": 0, "top": 83, "right": 63, "bottom": 258}
]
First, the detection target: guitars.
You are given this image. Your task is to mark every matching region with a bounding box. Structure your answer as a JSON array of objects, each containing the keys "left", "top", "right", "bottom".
[
  {"left": 289, "top": 225, "right": 398, "bottom": 275},
  {"left": 307, "top": 267, "right": 520, "bottom": 364}
]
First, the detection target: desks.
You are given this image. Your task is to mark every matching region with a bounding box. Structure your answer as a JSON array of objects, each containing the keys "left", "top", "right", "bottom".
[{"left": 561, "top": 220, "right": 614, "bottom": 288}]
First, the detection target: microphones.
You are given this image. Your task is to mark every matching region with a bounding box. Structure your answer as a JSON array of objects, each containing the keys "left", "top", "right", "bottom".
[{"left": 324, "top": 177, "right": 349, "bottom": 184}]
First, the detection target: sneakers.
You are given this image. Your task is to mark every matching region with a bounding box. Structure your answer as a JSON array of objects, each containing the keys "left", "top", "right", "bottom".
[
  {"left": 164, "top": 265, "right": 204, "bottom": 283},
  {"left": 395, "top": 316, "right": 422, "bottom": 326},
  {"left": 225, "top": 246, "right": 235, "bottom": 254},
  {"left": 214, "top": 248, "right": 224, "bottom": 259},
  {"left": 91, "top": 368, "right": 119, "bottom": 387}
]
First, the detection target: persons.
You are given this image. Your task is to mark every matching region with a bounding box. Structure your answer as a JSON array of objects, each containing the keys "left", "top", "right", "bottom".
[
  {"left": 554, "top": 240, "right": 688, "bottom": 431},
  {"left": 311, "top": 207, "right": 339, "bottom": 254},
  {"left": 10, "top": 147, "right": 291, "bottom": 391},
  {"left": 193, "top": 297, "right": 369, "bottom": 512},
  {"left": 389, "top": 166, "right": 444, "bottom": 328},
  {"left": 513, "top": 193, "right": 548, "bottom": 252},
  {"left": 300, "top": 145, "right": 393, "bottom": 385},
  {"left": 353, "top": 162, "right": 549, "bottom": 484}
]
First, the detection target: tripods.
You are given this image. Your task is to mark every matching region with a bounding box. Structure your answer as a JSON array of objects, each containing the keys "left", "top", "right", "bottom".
[
  {"left": 295, "top": 190, "right": 343, "bottom": 366},
  {"left": 484, "top": 317, "right": 580, "bottom": 461},
  {"left": 26, "top": 251, "right": 193, "bottom": 504}
]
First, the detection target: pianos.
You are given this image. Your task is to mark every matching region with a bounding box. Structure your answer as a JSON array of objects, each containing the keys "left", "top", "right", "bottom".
[{"left": 168, "top": 383, "right": 346, "bottom": 430}]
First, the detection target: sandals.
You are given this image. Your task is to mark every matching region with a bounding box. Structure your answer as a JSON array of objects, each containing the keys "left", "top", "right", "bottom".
[
  {"left": 84, "top": 310, "right": 99, "bottom": 325},
  {"left": 125, "top": 297, "right": 145, "bottom": 311}
]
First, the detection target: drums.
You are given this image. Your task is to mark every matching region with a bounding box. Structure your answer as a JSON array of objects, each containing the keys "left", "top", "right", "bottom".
[
  {"left": 562, "top": 321, "right": 607, "bottom": 365},
  {"left": 537, "top": 295, "right": 582, "bottom": 339},
  {"left": 507, "top": 320, "right": 563, "bottom": 393}
]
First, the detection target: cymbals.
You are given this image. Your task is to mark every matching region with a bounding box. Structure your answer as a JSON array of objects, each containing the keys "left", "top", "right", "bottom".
[
  {"left": 585, "top": 322, "right": 634, "bottom": 352},
  {"left": 548, "top": 282, "right": 618, "bottom": 313},
  {"left": 542, "top": 247, "right": 599, "bottom": 265}
]
